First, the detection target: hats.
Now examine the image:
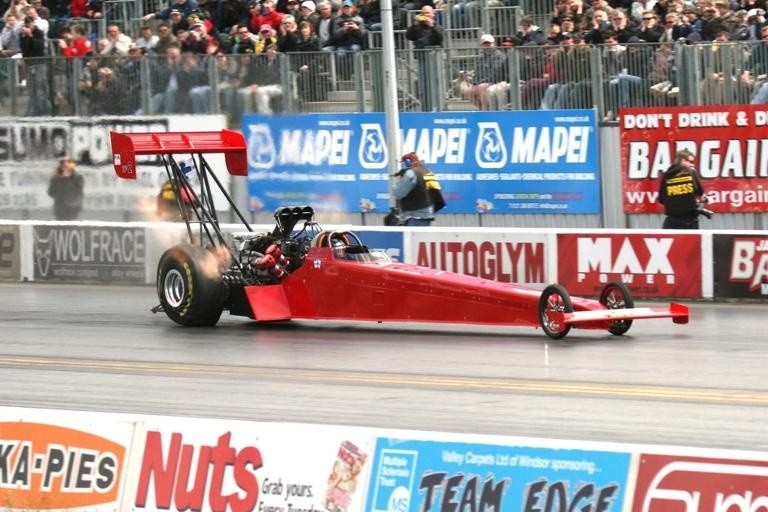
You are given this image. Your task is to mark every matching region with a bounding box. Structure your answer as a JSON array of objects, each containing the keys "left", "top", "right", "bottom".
[
  {"left": 559, "top": 31, "right": 573, "bottom": 40},
  {"left": 400, "top": 151, "right": 416, "bottom": 163},
  {"left": 261, "top": 24, "right": 271, "bottom": 33},
  {"left": 343, "top": 0, "right": 352, "bottom": 7},
  {"left": 479, "top": 34, "right": 495, "bottom": 46}
]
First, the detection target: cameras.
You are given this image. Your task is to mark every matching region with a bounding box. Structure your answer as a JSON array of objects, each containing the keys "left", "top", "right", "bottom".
[
  {"left": 417, "top": 15, "right": 427, "bottom": 21},
  {"left": 395, "top": 169, "right": 405, "bottom": 178},
  {"left": 189, "top": 30, "right": 195, "bottom": 35},
  {"left": 695, "top": 201, "right": 714, "bottom": 219}
]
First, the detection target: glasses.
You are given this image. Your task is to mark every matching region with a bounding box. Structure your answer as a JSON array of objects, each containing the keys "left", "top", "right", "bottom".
[{"left": 683, "top": 158, "right": 694, "bottom": 164}]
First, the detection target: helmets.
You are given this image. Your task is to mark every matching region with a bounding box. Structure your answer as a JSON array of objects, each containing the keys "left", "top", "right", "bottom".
[{"left": 325, "top": 238, "right": 348, "bottom": 261}]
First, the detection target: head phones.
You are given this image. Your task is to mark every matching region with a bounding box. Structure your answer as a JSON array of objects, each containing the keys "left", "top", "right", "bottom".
[{"left": 404, "top": 158, "right": 411, "bottom": 167}]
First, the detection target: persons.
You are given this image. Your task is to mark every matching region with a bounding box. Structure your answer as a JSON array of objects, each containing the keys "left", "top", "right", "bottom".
[
  {"left": 47, "top": 150, "right": 83, "bottom": 222},
  {"left": 655, "top": 147, "right": 707, "bottom": 229},
  {"left": 157, "top": 158, "right": 201, "bottom": 221},
  {"left": 389, "top": 152, "right": 446, "bottom": 227}
]
